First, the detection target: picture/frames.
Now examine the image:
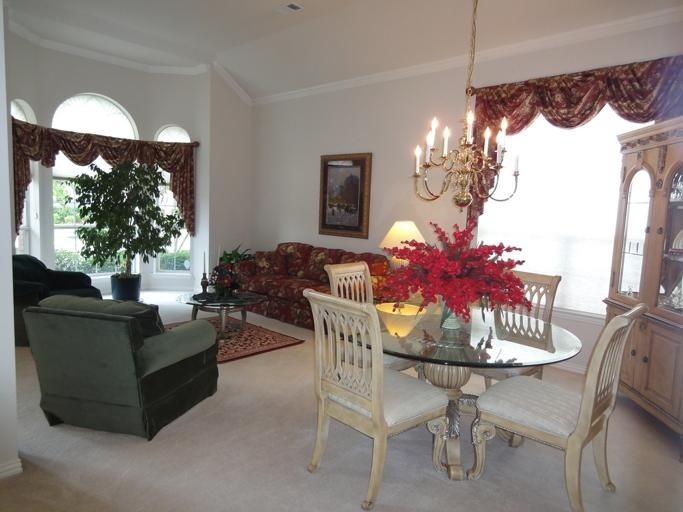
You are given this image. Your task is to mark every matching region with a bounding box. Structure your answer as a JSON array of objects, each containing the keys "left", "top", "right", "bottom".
[{"left": 319, "top": 149, "right": 371, "bottom": 239}]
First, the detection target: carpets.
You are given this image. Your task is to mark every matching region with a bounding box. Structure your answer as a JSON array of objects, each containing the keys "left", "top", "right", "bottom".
[{"left": 163, "top": 315, "right": 305, "bottom": 361}]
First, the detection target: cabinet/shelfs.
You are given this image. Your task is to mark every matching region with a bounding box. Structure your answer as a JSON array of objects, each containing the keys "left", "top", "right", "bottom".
[{"left": 603, "top": 116, "right": 683, "bottom": 435}]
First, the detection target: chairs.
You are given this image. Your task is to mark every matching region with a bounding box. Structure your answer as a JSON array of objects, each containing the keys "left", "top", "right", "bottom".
[
  {"left": 22, "top": 294, "right": 219, "bottom": 441},
  {"left": 12, "top": 254, "right": 101, "bottom": 347}
]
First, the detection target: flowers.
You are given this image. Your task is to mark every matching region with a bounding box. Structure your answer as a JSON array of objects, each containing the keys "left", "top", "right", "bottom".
[
  {"left": 209, "top": 245, "right": 258, "bottom": 298},
  {"left": 380, "top": 214, "right": 533, "bottom": 325}
]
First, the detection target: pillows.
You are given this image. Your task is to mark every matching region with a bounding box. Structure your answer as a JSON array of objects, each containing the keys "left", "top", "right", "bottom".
[{"left": 255, "top": 250, "right": 289, "bottom": 275}]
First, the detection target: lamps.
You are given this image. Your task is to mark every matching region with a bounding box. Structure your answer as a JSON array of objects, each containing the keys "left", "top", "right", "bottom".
[
  {"left": 408, "top": 0, "right": 521, "bottom": 213},
  {"left": 378, "top": 221, "right": 425, "bottom": 266}
]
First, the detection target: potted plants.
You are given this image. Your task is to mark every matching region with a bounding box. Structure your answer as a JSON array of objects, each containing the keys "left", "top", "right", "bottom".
[{"left": 63, "top": 154, "right": 185, "bottom": 301}]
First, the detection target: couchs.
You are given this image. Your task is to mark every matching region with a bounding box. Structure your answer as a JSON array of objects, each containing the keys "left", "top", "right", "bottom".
[{"left": 229, "top": 242, "right": 388, "bottom": 331}]
